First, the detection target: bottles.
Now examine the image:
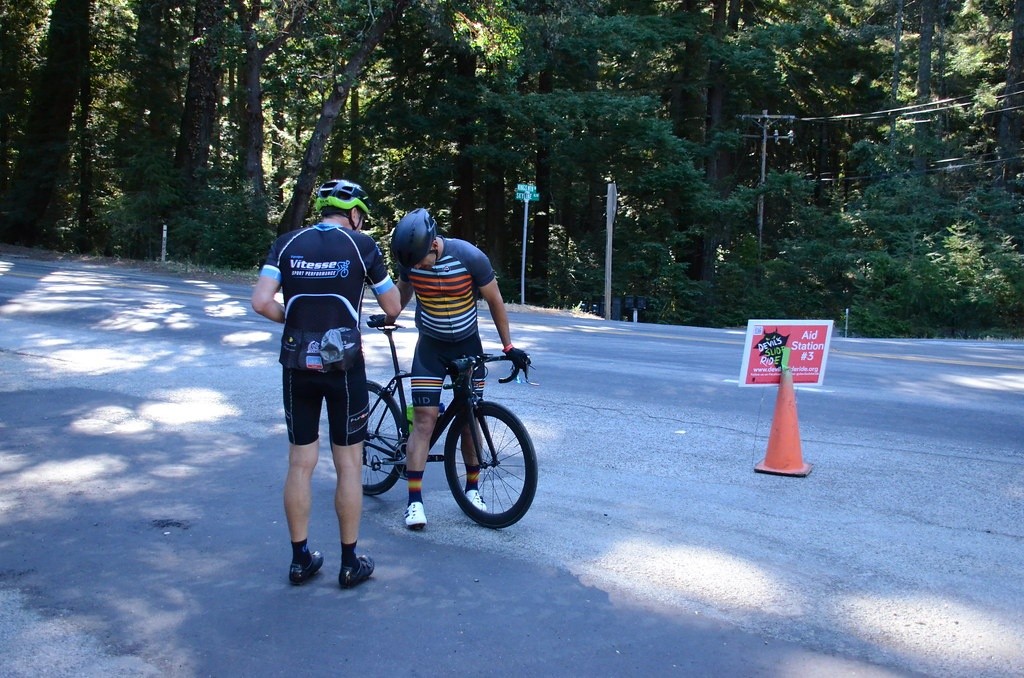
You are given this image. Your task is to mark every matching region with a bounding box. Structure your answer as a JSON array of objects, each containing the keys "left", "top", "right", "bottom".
[{"left": 406, "top": 402, "right": 414, "bottom": 432}]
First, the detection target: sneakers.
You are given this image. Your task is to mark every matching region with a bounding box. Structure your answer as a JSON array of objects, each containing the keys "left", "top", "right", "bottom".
[
  {"left": 289, "top": 550, "right": 324, "bottom": 586},
  {"left": 404, "top": 502, "right": 428, "bottom": 530},
  {"left": 464, "top": 489, "right": 487, "bottom": 512},
  {"left": 338, "top": 554, "right": 375, "bottom": 589}
]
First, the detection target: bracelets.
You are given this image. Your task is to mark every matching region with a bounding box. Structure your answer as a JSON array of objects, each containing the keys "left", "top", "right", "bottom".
[{"left": 502, "top": 343, "right": 513, "bottom": 353}]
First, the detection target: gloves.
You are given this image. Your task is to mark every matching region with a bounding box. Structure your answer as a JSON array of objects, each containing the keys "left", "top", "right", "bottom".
[
  {"left": 502, "top": 344, "right": 531, "bottom": 371},
  {"left": 366, "top": 314, "right": 387, "bottom": 328}
]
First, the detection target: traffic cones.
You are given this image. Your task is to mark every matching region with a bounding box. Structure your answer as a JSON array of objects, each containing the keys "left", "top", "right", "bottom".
[{"left": 754, "top": 366, "right": 814, "bottom": 477}]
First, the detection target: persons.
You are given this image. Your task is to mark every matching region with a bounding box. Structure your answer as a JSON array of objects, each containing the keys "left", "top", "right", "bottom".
[
  {"left": 251, "top": 181, "right": 400, "bottom": 588},
  {"left": 366, "top": 206, "right": 532, "bottom": 529}
]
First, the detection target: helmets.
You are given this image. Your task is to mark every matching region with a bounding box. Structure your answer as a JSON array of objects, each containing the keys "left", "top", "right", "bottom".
[
  {"left": 315, "top": 178, "right": 369, "bottom": 225},
  {"left": 390, "top": 207, "right": 438, "bottom": 269}
]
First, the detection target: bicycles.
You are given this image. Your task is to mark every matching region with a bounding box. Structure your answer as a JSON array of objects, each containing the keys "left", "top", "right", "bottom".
[{"left": 361, "top": 313, "right": 537, "bottom": 528}]
